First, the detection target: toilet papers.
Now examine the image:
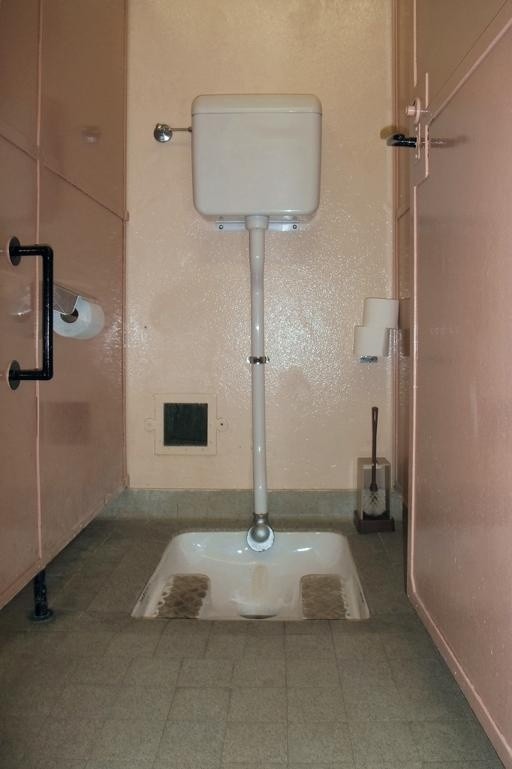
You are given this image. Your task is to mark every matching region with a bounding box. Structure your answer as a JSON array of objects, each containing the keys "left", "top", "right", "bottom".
[
  {"left": 354, "top": 298, "right": 398, "bottom": 359},
  {"left": 54, "top": 298, "right": 106, "bottom": 340}
]
